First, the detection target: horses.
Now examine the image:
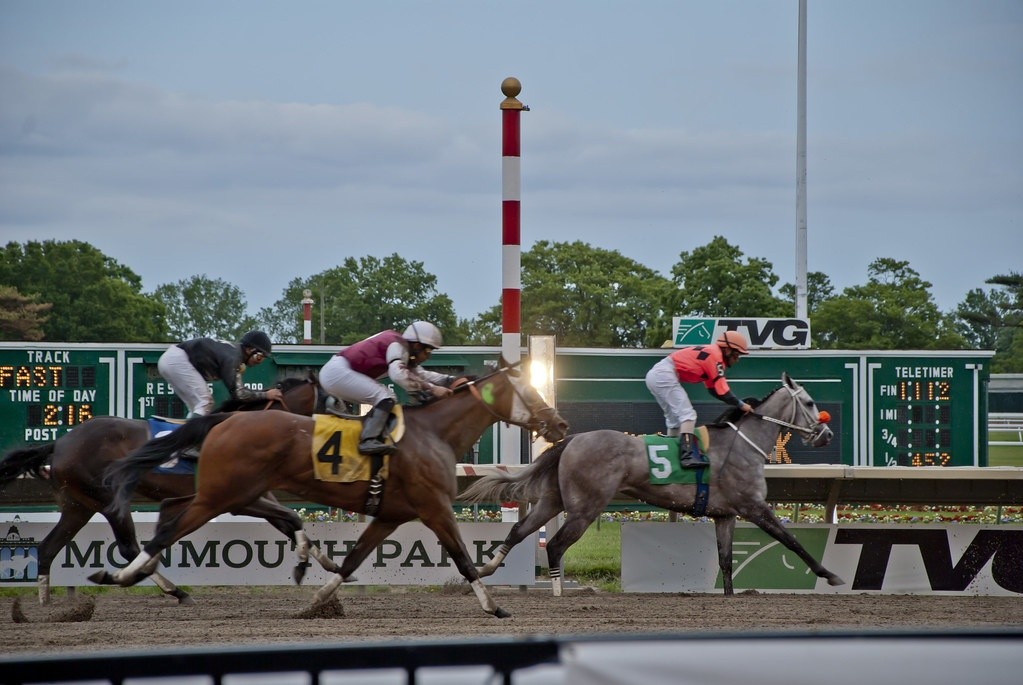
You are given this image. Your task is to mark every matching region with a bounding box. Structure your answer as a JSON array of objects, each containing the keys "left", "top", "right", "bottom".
[
  {"left": 87, "top": 357, "right": 571, "bottom": 621},
  {"left": 453, "top": 371, "right": 844, "bottom": 598},
  {"left": 0, "top": 370, "right": 351, "bottom": 607}
]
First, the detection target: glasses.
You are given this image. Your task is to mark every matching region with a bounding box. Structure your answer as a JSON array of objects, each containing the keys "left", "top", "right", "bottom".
[
  {"left": 726, "top": 350, "right": 740, "bottom": 358},
  {"left": 248, "top": 345, "right": 264, "bottom": 362},
  {"left": 416, "top": 344, "right": 432, "bottom": 353}
]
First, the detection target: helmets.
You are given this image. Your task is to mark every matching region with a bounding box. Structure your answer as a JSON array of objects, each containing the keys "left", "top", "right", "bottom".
[
  {"left": 241, "top": 330, "right": 272, "bottom": 356},
  {"left": 717, "top": 331, "right": 750, "bottom": 354},
  {"left": 402, "top": 320, "right": 442, "bottom": 350}
]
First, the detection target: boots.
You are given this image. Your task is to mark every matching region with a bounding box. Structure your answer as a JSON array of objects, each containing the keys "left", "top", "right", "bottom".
[
  {"left": 181, "top": 412, "right": 200, "bottom": 457},
  {"left": 358, "top": 408, "right": 397, "bottom": 453},
  {"left": 680, "top": 433, "right": 709, "bottom": 466}
]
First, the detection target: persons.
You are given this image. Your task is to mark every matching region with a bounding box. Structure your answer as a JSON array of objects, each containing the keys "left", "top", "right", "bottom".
[
  {"left": 158, "top": 331, "right": 285, "bottom": 458},
  {"left": 318, "top": 321, "right": 466, "bottom": 454},
  {"left": 645, "top": 331, "right": 757, "bottom": 470}
]
